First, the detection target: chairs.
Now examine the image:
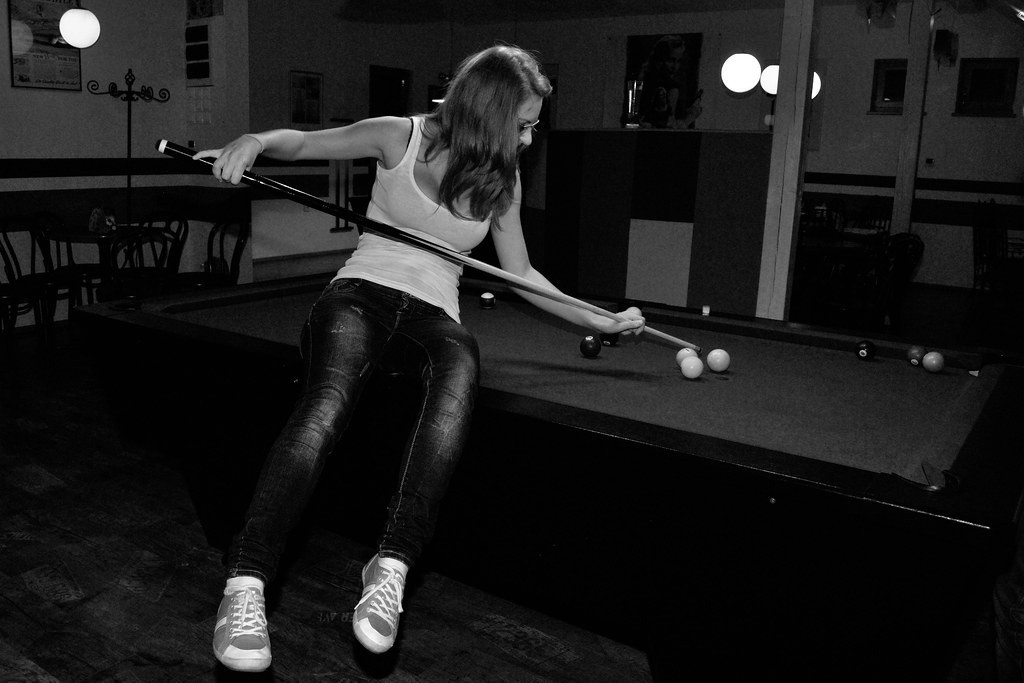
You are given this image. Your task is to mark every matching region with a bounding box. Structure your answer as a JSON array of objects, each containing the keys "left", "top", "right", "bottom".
[
  {"left": 806, "top": 193, "right": 926, "bottom": 335},
  {"left": 1, "top": 204, "right": 261, "bottom": 349}
]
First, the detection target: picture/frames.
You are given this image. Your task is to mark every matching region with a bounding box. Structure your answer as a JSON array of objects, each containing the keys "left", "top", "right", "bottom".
[
  {"left": 7, "top": 0, "right": 83, "bottom": 92},
  {"left": 288, "top": 69, "right": 323, "bottom": 128}
]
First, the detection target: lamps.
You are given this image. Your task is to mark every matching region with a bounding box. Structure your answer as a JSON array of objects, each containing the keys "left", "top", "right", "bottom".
[
  {"left": 58, "top": 1, "right": 101, "bottom": 49},
  {"left": 720, "top": 1, "right": 822, "bottom": 100}
]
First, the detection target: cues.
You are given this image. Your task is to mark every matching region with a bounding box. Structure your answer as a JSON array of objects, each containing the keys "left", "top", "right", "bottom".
[{"left": 154, "top": 135, "right": 704, "bottom": 354}]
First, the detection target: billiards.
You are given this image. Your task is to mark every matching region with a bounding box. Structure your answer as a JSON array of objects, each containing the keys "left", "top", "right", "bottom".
[
  {"left": 679, "top": 356, "right": 704, "bottom": 379},
  {"left": 921, "top": 351, "right": 946, "bottom": 374},
  {"left": 479, "top": 292, "right": 497, "bottom": 311},
  {"left": 579, "top": 335, "right": 602, "bottom": 359},
  {"left": 676, "top": 347, "right": 699, "bottom": 368},
  {"left": 906, "top": 344, "right": 929, "bottom": 367},
  {"left": 706, "top": 348, "right": 730, "bottom": 372},
  {"left": 598, "top": 331, "right": 620, "bottom": 347},
  {"left": 854, "top": 340, "right": 877, "bottom": 361}
]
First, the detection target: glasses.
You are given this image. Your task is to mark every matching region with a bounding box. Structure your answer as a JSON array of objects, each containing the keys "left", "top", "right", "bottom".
[{"left": 519, "top": 120, "right": 539, "bottom": 133}]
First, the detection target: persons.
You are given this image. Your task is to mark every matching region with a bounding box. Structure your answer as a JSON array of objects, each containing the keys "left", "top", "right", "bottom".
[
  {"left": 193, "top": 47, "right": 644, "bottom": 672},
  {"left": 623, "top": 35, "right": 691, "bottom": 128}
]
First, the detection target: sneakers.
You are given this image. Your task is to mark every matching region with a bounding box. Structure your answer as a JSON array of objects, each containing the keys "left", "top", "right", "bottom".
[
  {"left": 212, "top": 586, "right": 272, "bottom": 672},
  {"left": 352, "top": 553, "right": 406, "bottom": 654}
]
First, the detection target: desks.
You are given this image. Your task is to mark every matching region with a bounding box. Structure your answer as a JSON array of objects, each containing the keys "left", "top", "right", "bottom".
[
  {"left": 73, "top": 273, "right": 1013, "bottom": 683},
  {"left": 802, "top": 233, "right": 879, "bottom": 271}
]
